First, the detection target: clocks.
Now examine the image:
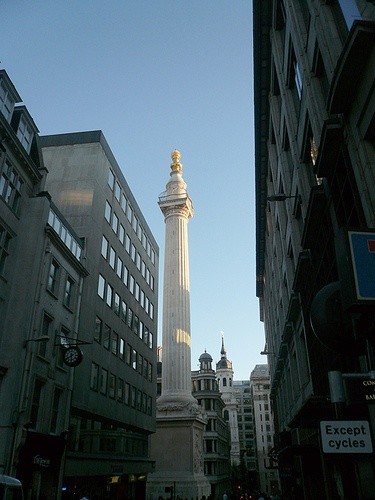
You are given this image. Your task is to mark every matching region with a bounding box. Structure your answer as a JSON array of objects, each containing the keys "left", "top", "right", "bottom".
[{"left": 54, "top": 335, "right": 93, "bottom": 367}]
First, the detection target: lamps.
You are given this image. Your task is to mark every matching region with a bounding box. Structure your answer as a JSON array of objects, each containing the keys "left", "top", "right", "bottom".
[{"left": 21, "top": 336, "right": 51, "bottom": 351}]
